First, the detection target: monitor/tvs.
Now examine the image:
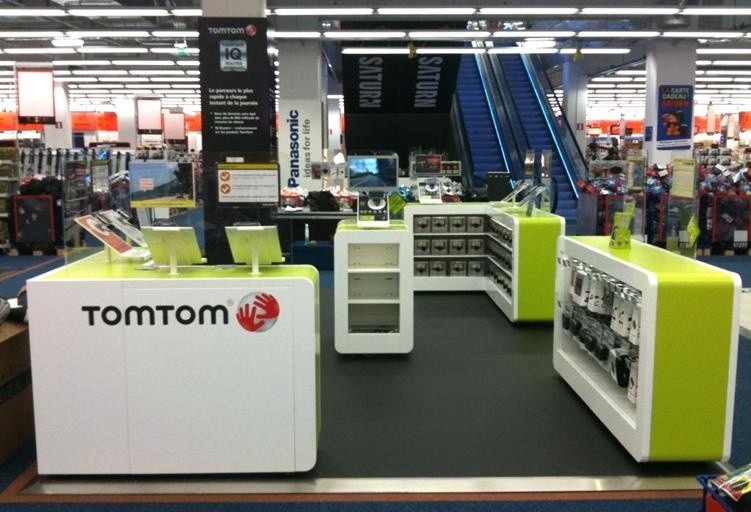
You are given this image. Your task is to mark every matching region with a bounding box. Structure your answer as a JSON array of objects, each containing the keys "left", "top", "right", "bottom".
[
  {"left": 348, "top": 155, "right": 399, "bottom": 191},
  {"left": 414, "top": 154, "right": 441, "bottom": 175}
]
